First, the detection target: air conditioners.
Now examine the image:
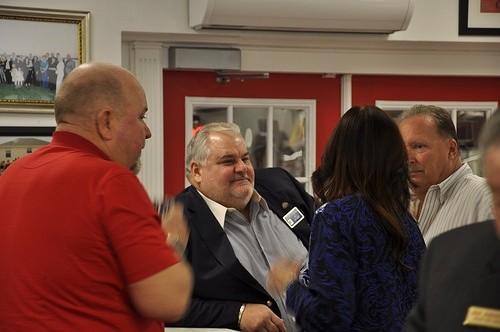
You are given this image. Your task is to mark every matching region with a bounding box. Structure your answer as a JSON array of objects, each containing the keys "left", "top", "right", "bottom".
[{"left": 188, "top": 0, "right": 413, "bottom": 36}]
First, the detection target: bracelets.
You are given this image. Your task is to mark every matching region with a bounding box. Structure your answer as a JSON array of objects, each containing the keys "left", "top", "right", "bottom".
[
  {"left": 238, "top": 303, "right": 245, "bottom": 324},
  {"left": 165, "top": 231, "right": 185, "bottom": 257}
]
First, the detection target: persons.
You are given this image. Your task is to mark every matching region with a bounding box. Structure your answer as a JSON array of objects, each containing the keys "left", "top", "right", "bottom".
[
  {"left": 0, "top": 52, "right": 75, "bottom": 93},
  {"left": 396, "top": 104, "right": 500, "bottom": 332},
  {"left": 159, "top": 123, "right": 319, "bottom": 332},
  {"left": 0, "top": 61, "right": 192, "bottom": 332},
  {"left": 266, "top": 106, "right": 426, "bottom": 332}
]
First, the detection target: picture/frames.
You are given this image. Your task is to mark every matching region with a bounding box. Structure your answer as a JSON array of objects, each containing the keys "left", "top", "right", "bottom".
[
  {"left": 0, "top": 126, "right": 56, "bottom": 175},
  {"left": 0, "top": 5, "right": 91, "bottom": 115},
  {"left": 458, "top": 0, "right": 500, "bottom": 36}
]
